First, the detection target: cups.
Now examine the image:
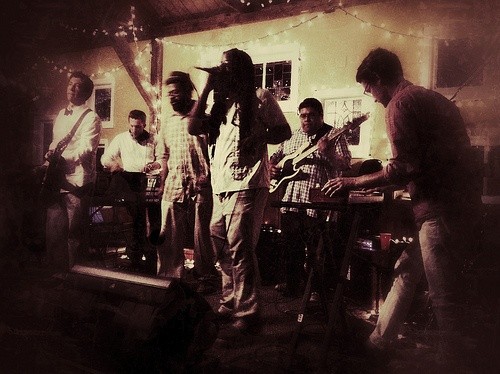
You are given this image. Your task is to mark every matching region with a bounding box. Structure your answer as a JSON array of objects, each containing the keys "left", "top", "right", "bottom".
[{"left": 380, "top": 232, "right": 391, "bottom": 250}]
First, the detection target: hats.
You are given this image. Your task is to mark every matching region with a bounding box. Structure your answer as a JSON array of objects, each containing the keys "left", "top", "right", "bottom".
[{"left": 298, "top": 98, "right": 322, "bottom": 112}]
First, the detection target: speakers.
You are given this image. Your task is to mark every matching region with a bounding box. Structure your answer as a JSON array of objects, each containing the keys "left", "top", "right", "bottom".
[{"left": 53, "top": 265, "right": 220, "bottom": 369}]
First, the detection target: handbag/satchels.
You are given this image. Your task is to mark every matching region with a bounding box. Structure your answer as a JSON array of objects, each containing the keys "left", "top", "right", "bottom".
[{"left": 26, "top": 162, "right": 65, "bottom": 202}]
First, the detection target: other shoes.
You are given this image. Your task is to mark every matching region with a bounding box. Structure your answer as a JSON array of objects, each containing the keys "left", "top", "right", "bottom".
[
  {"left": 196, "top": 276, "right": 221, "bottom": 295},
  {"left": 218, "top": 317, "right": 269, "bottom": 340},
  {"left": 275, "top": 277, "right": 296, "bottom": 293},
  {"left": 211, "top": 303, "right": 238, "bottom": 320},
  {"left": 310, "top": 289, "right": 321, "bottom": 302}
]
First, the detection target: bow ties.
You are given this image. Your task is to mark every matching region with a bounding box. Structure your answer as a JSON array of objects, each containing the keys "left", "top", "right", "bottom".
[{"left": 64, "top": 108, "right": 73, "bottom": 116}]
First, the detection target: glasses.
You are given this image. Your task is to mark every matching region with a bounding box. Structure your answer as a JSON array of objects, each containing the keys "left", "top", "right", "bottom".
[
  {"left": 363, "top": 80, "right": 375, "bottom": 97},
  {"left": 299, "top": 113, "right": 321, "bottom": 119}
]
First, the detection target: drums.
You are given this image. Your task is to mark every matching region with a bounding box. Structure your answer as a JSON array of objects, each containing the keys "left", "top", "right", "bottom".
[{"left": 146, "top": 174, "right": 162, "bottom": 207}]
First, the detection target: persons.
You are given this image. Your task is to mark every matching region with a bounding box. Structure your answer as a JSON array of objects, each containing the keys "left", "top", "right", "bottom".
[
  {"left": 321, "top": 47, "right": 470, "bottom": 353},
  {"left": 40, "top": 71, "right": 101, "bottom": 269},
  {"left": 144, "top": 71, "right": 218, "bottom": 294},
  {"left": 268, "top": 98, "right": 351, "bottom": 304},
  {"left": 187, "top": 47, "right": 291, "bottom": 344},
  {"left": 101, "top": 110, "right": 161, "bottom": 252}
]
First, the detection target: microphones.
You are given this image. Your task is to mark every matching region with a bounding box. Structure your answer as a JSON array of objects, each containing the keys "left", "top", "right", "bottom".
[{"left": 194, "top": 66, "right": 222, "bottom": 75}]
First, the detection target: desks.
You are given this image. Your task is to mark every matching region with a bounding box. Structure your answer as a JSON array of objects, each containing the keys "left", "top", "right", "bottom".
[{"left": 261, "top": 200, "right": 386, "bottom": 373}]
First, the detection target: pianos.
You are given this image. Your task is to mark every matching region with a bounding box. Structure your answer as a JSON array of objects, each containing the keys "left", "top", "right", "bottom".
[{"left": 283, "top": 188, "right": 384, "bottom": 374}]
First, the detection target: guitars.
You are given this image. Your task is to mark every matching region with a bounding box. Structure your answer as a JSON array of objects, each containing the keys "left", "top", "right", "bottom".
[
  {"left": 268, "top": 111, "right": 370, "bottom": 194},
  {"left": 34, "top": 133, "right": 73, "bottom": 209}
]
